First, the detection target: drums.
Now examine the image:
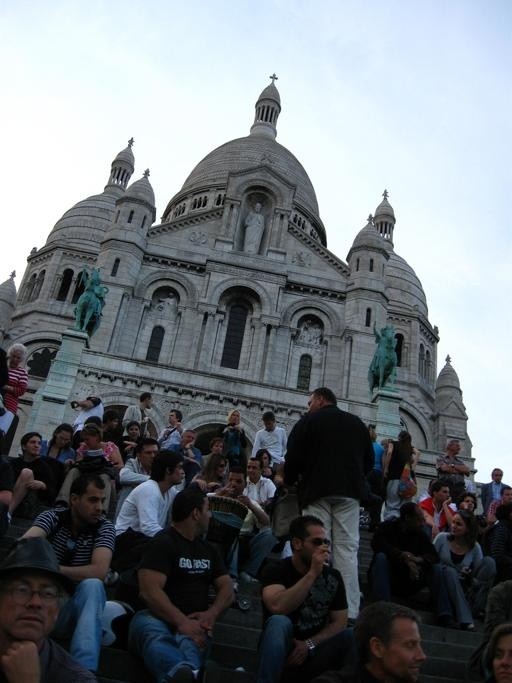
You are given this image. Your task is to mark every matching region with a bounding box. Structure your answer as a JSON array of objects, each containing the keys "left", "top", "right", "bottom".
[{"left": 207, "top": 491, "right": 249, "bottom": 544}]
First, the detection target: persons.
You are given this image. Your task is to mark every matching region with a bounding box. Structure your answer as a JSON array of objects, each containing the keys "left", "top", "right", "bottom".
[
  {"left": 311, "top": 601, "right": 426, "bottom": 682},
  {"left": 368, "top": 429, "right": 510, "bottom": 682},
  {"left": 243, "top": 200, "right": 265, "bottom": 254},
  {"left": 1, "top": 343, "right": 28, "bottom": 456},
  {"left": 255, "top": 515, "right": 355, "bottom": 681},
  {"left": 0, "top": 392, "right": 288, "bottom": 681},
  {"left": 285, "top": 388, "right": 374, "bottom": 628}
]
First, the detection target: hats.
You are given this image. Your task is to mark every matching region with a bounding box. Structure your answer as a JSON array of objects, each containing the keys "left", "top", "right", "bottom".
[{"left": 0, "top": 537, "right": 75, "bottom": 593}]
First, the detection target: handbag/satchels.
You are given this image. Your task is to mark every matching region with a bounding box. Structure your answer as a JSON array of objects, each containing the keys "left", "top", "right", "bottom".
[
  {"left": 271, "top": 494, "right": 301, "bottom": 538},
  {"left": 398, "top": 465, "right": 417, "bottom": 498}
]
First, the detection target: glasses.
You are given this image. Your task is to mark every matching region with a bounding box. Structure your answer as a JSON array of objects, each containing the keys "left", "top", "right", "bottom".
[
  {"left": 12, "top": 585, "right": 59, "bottom": 606},
  {"left": 304, "top": 538, "right": 331, "bottom": 547}
]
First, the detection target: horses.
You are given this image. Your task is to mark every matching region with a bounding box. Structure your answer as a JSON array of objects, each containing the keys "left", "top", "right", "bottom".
[
  {"left": 367, "top": 325, "right": 397, "bottom": 400},
  {"left": 74, "top": 269, "right": 103, "bottom": 338}
]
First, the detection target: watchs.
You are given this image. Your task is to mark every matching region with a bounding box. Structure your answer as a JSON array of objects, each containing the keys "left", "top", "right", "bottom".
[{"left": 305, "top": 639, "right": 316, "bottom": 653}]
